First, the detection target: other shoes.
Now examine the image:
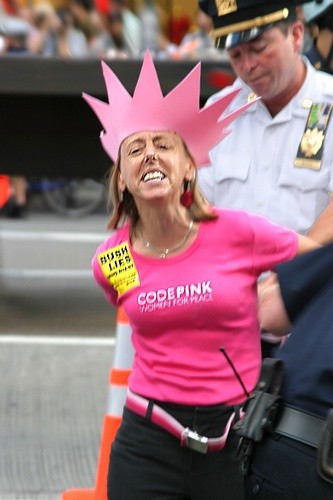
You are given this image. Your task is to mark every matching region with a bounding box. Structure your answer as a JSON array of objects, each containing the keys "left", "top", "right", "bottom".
[{"left": 1, "top": 198, "right": 27, "bottom": 219}]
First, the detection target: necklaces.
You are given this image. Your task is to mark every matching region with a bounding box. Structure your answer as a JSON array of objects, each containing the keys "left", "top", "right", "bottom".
[{"left": 138, "top": 213, "right": 196, "bottom": 258}]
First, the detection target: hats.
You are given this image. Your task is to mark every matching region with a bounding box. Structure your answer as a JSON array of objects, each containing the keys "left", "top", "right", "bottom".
[
  {"left": 198, "top": 0, "right": 296, "bottom": 49},
  {"left": 304, "top": 0, "right": 333, "bottom": 27}
]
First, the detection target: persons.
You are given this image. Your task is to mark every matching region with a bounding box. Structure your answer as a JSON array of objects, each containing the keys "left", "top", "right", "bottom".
[
  {"left": 1, "top": 1, "right": 333, "bottom": 72},
  {"left": 91, "top": 109, "right": 323, "bottom": 500},
  {"left": 192, "top": 0, "right": 333, "bottom": 246},
  {"left": 245, "top": 239, "right": 333, "bottom": 500},
  {"left": 0, "top": 18, "right": 34, "bottom": 218}
]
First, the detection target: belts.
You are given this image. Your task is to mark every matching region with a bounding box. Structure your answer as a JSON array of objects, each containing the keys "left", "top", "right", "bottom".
[
  {"left": 272, "top": 404, "right": 327, "bottom": 448},
  {"left": 126, "top": 389, "right": 246, "bottom": 454}
]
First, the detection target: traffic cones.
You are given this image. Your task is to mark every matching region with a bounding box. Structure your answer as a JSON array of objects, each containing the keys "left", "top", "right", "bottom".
[{"left": 59, "top": 305, "right": 134, "bottom": 500}]
